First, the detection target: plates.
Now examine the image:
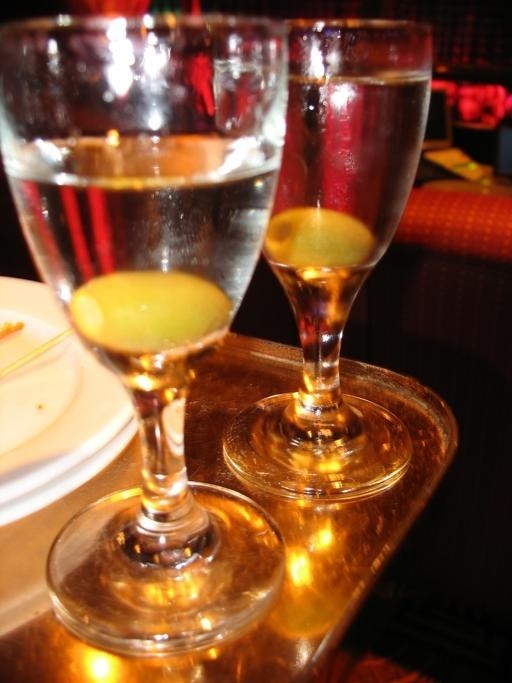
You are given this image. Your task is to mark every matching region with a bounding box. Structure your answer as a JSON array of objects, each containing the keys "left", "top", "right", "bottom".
[{"left": 0, "top": 275, "right": 141, "bottom": 531}]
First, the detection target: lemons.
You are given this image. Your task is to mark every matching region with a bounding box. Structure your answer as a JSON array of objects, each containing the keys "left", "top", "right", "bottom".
[
  {"left": 265, "top": 208, "right": 375, "bottom": 266},
  {"left": 70, "top": 269, "right": 231, "bottom": 353}
]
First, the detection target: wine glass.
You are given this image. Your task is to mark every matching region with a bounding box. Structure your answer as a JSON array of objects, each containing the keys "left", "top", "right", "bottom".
[
  {"left": 221, "top": 16, "right": 436, "bottom": 503},
  {"left": 0, "top": 12, "right": 299, "bottom": 660}
]
